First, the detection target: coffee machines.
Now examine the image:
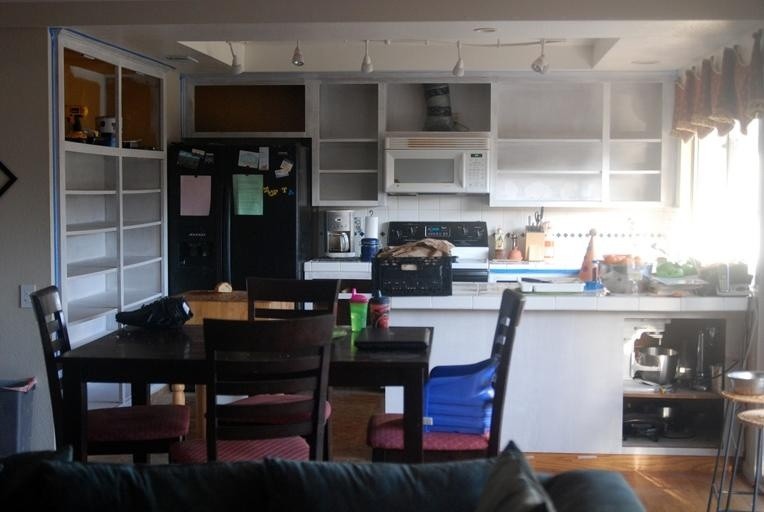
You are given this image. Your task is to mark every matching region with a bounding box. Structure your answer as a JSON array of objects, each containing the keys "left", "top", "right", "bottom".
[{"left": 323, "top": 208, "right": 356, "bottom": 259}]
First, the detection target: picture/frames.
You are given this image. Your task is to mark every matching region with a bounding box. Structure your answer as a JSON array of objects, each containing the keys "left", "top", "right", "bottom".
[{"left": 0, "top": 159, "right": 19, "bottom": 198}]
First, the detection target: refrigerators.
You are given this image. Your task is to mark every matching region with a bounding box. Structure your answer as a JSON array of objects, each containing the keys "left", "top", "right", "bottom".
[{"left": 163, "top": 138, "right": 313, "bottom": 290}]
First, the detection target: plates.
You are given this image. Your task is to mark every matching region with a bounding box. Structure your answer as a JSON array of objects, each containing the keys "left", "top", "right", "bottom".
[{"left": 648, "top": 271, "right": 708, "bottom": 287}]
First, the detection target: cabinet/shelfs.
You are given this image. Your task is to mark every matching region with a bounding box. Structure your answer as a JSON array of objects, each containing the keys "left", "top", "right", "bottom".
[
  {"left": 311, "top": 80, "right": 388, "bottom": 209},
  {"left": 490, "top": 74, "right": 678, "bottom": 210},
  {"left": 182, "top": 77, "right": 314, "bottom": 137},
  {"left": 56, "top": 30, "right": 167, "bottom": 352},
  {"left": 617, "top": 311, "right": 733, "bottom": 454}
]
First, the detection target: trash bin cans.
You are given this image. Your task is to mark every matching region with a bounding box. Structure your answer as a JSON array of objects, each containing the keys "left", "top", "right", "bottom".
[{"left": 1, "top": 378, "right": 36, "bottom": 455}]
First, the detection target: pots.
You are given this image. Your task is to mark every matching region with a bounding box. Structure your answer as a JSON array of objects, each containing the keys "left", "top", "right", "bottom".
[{"left": 623, "top": 404, "right": 682, "bottom": 442}]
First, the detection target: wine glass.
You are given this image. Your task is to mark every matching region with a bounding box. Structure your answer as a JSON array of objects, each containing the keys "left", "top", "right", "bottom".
[{"left": 623, "top": 259, "right": 645, "bottom": 296}]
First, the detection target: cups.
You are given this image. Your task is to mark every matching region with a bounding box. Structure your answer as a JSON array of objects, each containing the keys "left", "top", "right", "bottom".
[
  {"left": 370, "top": 289, "right": 391, "bottom": 330},
  {"left": 348, "top": 288, "right": 368, "bottom": 332},
  {"left": 350, "top": 333, "right": 369, "bottom": 360}
]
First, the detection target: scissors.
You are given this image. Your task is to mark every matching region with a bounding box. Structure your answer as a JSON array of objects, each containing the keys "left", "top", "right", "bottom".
[{"left": 534, "top": 211, "right": 542, "bottom": 233}]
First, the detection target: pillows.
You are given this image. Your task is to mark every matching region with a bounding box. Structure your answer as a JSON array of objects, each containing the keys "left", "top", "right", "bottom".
[{"left": 475, "top": 441, "right": 560, "bottom": 512}]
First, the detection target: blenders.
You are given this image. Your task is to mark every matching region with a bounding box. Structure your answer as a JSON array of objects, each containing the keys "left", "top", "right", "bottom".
[{"left": 623, "top": 318, "right": 684, "bottom": 392}]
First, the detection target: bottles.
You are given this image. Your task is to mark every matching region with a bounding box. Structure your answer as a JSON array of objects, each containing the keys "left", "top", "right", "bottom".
[
  {"left": 361, "top": 238, "right": 378, "bottom": 261},
  {"left": 493, "top": 228, "right": 506, "bottom": 259}
]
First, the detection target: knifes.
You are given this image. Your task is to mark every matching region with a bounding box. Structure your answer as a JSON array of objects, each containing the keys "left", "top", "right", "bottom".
[{"left": 527, "top": 205, "right": 545, "bottom": 232}]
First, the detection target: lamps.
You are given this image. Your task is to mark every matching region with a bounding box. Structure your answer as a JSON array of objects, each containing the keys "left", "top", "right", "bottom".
[{"left": 218, "top": 40, "right": 568, "bottom": 79}]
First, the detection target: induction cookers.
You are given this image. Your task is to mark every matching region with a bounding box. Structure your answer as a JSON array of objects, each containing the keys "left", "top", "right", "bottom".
[{"left": 451, "top": 256, "right": 488, "bottom": 271}]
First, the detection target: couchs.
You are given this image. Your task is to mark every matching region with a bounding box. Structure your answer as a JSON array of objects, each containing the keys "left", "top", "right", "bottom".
[{"left": 0, "top": 441, "right": 648, "bottom": 511}]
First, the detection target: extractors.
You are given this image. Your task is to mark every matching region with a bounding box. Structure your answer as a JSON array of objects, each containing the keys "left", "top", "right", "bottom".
[{"left": 383, "top": 82, "right": 491, "bottom": 148}]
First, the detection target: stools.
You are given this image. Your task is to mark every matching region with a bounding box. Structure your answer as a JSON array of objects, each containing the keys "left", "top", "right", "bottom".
[
  {"left": 723, "top": 407, "right": 764, "bottom": 512},
  {"left": 705, "top": 389, "right": 764, "bottom": 512}
]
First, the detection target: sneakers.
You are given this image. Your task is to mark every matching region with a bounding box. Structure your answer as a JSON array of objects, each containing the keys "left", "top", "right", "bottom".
[{"left": 116, "top": 297, "right": 193, "bottom": 328}]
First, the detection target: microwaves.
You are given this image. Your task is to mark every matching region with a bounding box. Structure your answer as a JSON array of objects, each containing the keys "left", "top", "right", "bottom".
[{"left": 383, "top": 148, "right": 490, "bottom": 194}]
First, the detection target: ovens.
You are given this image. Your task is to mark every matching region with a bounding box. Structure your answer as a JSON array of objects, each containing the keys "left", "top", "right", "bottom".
[{"left": 450, "top": 270, "right": 488, "bottom": 282}]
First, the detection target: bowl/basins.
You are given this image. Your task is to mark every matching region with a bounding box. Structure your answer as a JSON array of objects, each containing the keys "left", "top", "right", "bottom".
[
  {"left": 724, "top": 369, "right": 764, "bottom": 396},
  {"left": 597, "top": 259, "right": 654, "bottom": 296}
]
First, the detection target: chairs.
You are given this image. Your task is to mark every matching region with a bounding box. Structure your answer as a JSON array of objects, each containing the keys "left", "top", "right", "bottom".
[
  {"left": 200, "top": 311, "right": 337, "bottom": 464},
  {"left": 368, "top": 286, "right": 528, "bottom": 461},
  {"left": 29, "top": 285, "right": 195, "bottom": 466},
  {"left": 221, "top": 275, "right": 343, "bottom": 462}
]
